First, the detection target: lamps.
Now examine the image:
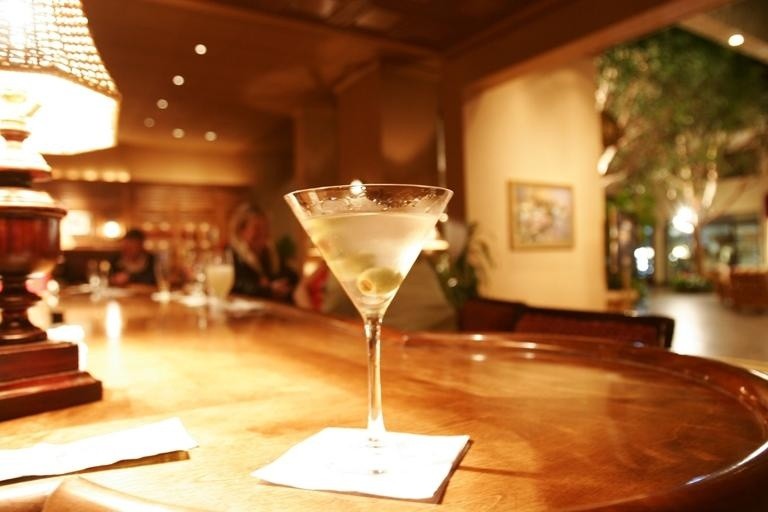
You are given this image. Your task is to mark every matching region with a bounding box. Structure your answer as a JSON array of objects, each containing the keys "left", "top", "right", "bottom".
[{"left": 0, "top": 0, "right": 121, "bottom": 422}]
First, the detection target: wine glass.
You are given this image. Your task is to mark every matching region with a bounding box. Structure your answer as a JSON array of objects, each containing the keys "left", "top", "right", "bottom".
[{"left": 283, "top": 183, "right": 455, "bottom": 471}]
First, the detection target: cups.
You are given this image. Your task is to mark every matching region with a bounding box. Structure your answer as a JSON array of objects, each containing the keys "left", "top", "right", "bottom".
[{"left": 206, "top": 249, "right": 235, "bottom": 300}]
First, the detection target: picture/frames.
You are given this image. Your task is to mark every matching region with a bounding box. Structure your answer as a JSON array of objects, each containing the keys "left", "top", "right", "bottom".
[{"left": 509, "top": 181, "right": 577, "bottom": 251}]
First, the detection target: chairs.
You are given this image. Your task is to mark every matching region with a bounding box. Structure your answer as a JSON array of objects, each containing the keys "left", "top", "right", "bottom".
[{"left": 458, "top": 296, "right": 674, "bottom": 357}]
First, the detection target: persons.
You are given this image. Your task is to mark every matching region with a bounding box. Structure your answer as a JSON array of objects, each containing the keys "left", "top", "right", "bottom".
[
  {"left": 110, "top": 228, "right": 153, "bottom": 287},
  {"left": 222, "top": 202, "right": 293, "bottom": 305}
]
